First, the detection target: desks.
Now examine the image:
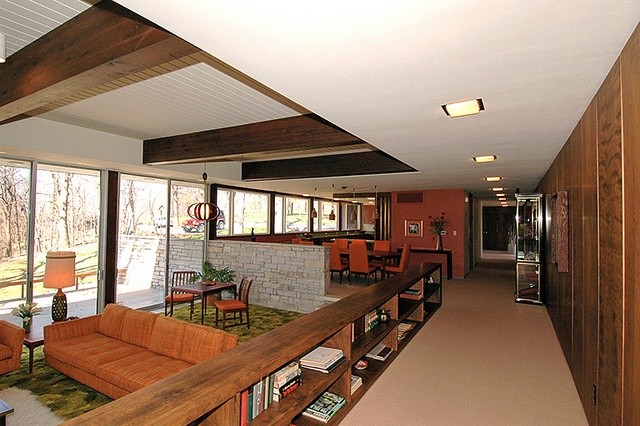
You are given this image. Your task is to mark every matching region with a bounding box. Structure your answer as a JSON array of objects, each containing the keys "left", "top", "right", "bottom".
[
  {"left": 23, "top": 325, "right": 44, "bottom": 373},
  {"left": 170, "top": 282, "right": 237, "bottom": 325},
  {"left": 397, "top": 247, "right": 452, "bottom": 280}
]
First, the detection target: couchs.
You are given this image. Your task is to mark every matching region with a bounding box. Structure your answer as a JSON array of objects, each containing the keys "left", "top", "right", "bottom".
[
  {"left": 43, "top": 303, "right": 238, "bottom": 400},
  {"left": 0, "top": 319, "right": 26, "bottom": 374}
]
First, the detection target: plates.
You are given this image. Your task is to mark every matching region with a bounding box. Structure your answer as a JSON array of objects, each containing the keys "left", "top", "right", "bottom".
[{"left": 202, "top": 280, "right": 217, "bottom": 286}]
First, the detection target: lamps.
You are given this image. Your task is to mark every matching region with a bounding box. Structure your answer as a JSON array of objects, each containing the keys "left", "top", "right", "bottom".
[
  {"left": 187, "top": 163, "right": 220, "bottom": 222},
  {"left": 373, "top": 184, "right": 379, "bottom": 219},
  {"left": 311, "top": 188, "right": 317, "bottom": 218},
  {"left": 351, "top": 188, "right": 357, "bottom": 220},
  {"left": 43, "top": 251, "right": 75, "bottom": 323},
  {"left": 329, "top": 184, "right": 335, "bottom": 220}
]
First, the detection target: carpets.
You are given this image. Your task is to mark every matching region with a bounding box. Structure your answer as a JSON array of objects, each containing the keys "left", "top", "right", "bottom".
[{"left": 0, "top": 386, "right": 64, "bottom": 426}]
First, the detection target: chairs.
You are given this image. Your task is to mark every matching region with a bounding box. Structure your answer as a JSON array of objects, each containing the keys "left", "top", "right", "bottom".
[
  {"left": 352, "top": 239, "right": 366, "bottom": 244},
  {"left": 349, "top": 243, "right": 377, "bottom": 286},
  {"left": 165, "top": 271, "right": 195, "bottom": 320},
  {"left": 322, "top": 242, "right": 349, "bottom": 284},
  {"left": 215, "top": 277, "right": 253, "bottom": 331},
  {"left": 292, "top": 239, "right": 301, "bottom": 244},
  {"left": 370, "top": 240, "right": 390, "bottom": 264},
  {"left": 299, "top": 240, "right": 314, "bottom": 245},
  {"left": 334, "top": 239, "right": 348, "bottom": 261},
  {"left": 381, "top": 242, "right": 411, "bottom": 281}
]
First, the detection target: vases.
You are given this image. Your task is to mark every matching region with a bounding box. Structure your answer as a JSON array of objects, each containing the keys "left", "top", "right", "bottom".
[
  {"left": 23, "top": 320, "right": 30, "bottom": 333},
  {"left": 435, "top": 235, "right": 443, "bottom": 250}
]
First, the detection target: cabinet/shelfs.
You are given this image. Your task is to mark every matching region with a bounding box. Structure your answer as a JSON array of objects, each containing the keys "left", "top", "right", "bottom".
[
  {"left": 239, "top": 321, "right": 351, "bottom": 426},
  {"left": 515, "top": 193, "right": 543, "bottom": 304},
  {"left": 351, "top": 292, "right": 398, "bottom": 409},
  {"left": 424, "top": 263, "right": 443, "bottom": 325},
  {"left": 399, "top": 273, "right": 424, "bottom": 354},
  {"left": 60, "top": 391, "right": 239, "bottom": 426}
]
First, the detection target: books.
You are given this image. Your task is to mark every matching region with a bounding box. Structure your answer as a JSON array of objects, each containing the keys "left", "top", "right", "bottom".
[
  {"left": 399, "top": 289, "right": 422, "bottom": 301},
  {"left": 351, "top": 309, "right": 379, "bottom": 343},
  {"left": 240, "top": 362, "right": 302, "bottom": 426},
  {"left": 300, "top": 346, "right": 347, "bottom": 373},
  {"left": 351, "top": 375, "right": 363, "bottom": 395},
  {"left": 365, "top": 343, "right": 393, "bottom": 361},
  {"left": 301, "top": 392, "right": 345, "bottom": 424}
]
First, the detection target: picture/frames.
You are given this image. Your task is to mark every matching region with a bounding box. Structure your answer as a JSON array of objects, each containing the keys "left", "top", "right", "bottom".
[
  {"left": 550, "top": 190, "right": 568, "bottom": 273},
  {"left": 405, "top": 219, "right": 423, "bottom": 238}
]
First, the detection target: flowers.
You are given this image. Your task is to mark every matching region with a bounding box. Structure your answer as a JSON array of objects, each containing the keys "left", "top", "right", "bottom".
[
  {"left": 11, "top": 302, "right": 43, "bottom": 326},
  {"left": 427, "top": 210, "right": 449, "bottom": 240}
]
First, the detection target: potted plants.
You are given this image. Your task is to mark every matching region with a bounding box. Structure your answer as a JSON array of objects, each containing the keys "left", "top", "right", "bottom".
[{"left": 190, "top": 261, "right": 236, "bottom": 305}]
